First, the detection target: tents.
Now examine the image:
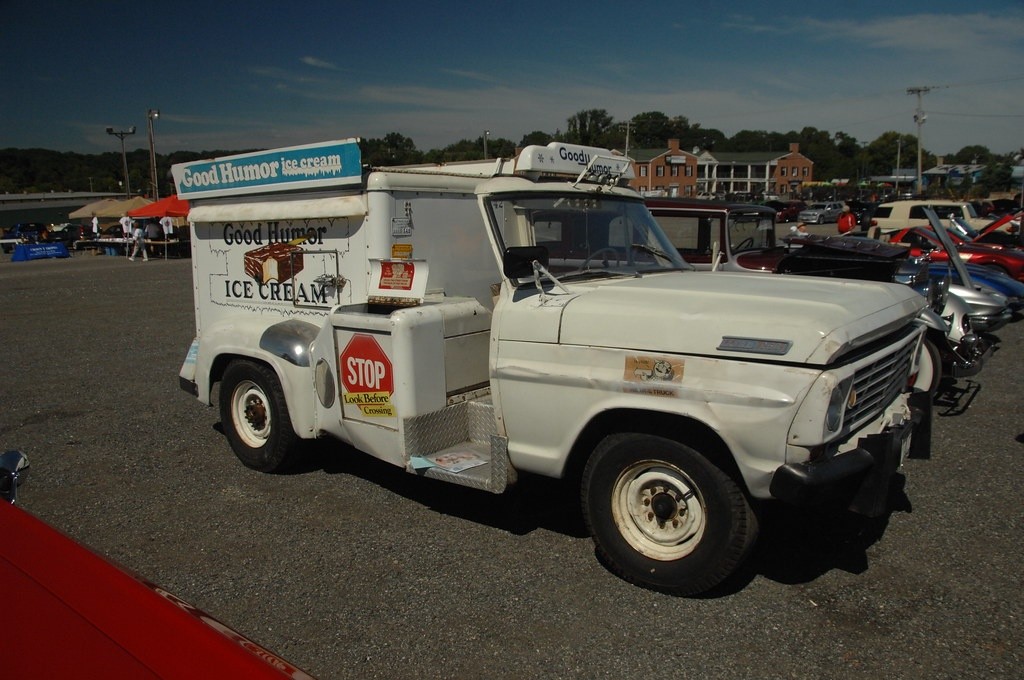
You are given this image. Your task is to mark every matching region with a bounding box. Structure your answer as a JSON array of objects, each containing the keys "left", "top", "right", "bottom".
[{"left": 68, "top": 195, "right": 191, "bottom": 260}]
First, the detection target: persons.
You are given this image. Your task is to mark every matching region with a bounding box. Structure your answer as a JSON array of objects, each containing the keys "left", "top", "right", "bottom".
[
  {"left": 797, "top": 223, "right": 808, "bottom": 233},
  {"left": 128, "top": 222, "right": 148, "bottom": 262}
]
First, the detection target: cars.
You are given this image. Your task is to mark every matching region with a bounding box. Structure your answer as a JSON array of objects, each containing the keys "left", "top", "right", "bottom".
[
  {"left": 525, "top": 194, "right": 1024, "bottom": 367},
  {"left": 46, "top": 224, "right": 102, "bottom": 249},
  {"left": 100, "top": 225, "right": 124, "bottom": 239}
]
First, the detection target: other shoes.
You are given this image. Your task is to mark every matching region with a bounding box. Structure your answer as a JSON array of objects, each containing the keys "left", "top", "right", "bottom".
[
  {"left": 129, "top": 257, "right": 135, "bottom": 261},
  {"left": 142, "top": 259, "right": 149, "bottom": 261}
]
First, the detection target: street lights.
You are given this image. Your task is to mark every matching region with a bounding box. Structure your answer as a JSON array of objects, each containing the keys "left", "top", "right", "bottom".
[
  {"left": 105, "top": 126, "right": 137, "bottom": 199},
  {"left": 147, "top": 108, "right": 161, "bottom": 202}
]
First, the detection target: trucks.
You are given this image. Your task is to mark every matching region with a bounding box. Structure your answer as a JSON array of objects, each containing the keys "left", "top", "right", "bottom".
[{"left": 171, "top": 139, "right": 951, "bottom": 599}]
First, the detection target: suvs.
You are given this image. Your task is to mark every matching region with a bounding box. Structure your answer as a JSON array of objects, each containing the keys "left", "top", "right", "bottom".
[{"left": 2, "top": 222, "right": 50, "bottom": 254}]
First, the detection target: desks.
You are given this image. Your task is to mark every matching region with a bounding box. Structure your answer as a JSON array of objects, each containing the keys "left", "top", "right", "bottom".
[{"left": 76, "top": 240, "right": 180, "bottom": 257}]
